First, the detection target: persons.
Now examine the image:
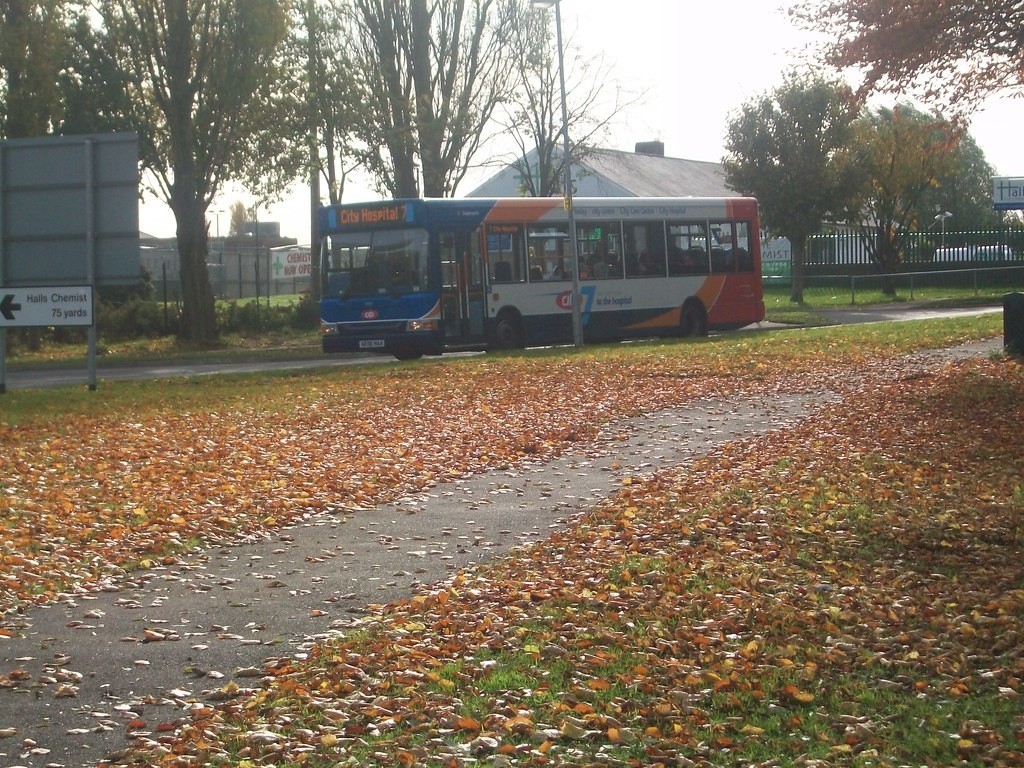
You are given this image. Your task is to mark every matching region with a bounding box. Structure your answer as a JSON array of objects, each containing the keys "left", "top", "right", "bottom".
[{"left": 527, "top": 237, "right": 753, "bottom": 281}]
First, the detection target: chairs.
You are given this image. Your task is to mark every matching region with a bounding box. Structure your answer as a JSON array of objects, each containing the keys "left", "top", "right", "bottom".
[{"left": 493, "top": 245, "right": 750, "bottom": 284}]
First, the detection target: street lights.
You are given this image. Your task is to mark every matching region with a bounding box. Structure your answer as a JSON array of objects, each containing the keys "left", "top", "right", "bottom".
[{"left": 208, "top": 210, "right": 224, "bottom": 245}]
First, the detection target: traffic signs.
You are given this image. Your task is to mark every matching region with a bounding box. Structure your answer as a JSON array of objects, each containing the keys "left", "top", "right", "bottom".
[{"left": 0, "top": 284, "right": 93, "bottom": 329}]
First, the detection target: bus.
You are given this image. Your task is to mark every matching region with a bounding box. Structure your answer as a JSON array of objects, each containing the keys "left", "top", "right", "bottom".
[{"left": 317, "top": 195, "right": 765, "bottom": 363}]
